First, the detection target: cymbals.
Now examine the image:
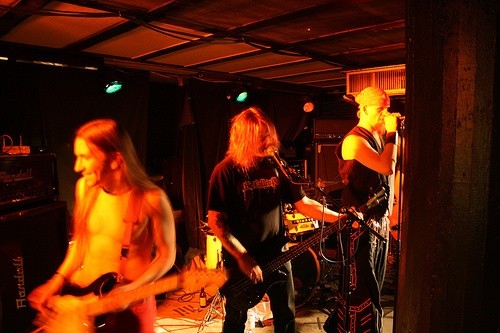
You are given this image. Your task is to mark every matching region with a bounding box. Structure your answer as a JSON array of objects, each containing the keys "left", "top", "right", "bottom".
[{"left": 305, "top": 182, "right": 337, "bottom": 193}]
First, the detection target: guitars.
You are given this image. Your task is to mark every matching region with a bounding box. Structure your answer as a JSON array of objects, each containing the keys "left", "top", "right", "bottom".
[
  {"left": 34, "top": 266, "right": 229, "bottom": 333},
  {"left": 216, "top": 185, "right": 390, "bottom": 311}
]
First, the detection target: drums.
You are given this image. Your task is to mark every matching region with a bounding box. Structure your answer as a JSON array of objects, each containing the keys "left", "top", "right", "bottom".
[
  {"left": 285, "top": 211, "right": 319, "bottom": 236},
  {"left": 204, "top": 232, "right": 223, "bottom": 269},
  {"left": 287, "top": 243, "right": 320, "bottom": 309}
]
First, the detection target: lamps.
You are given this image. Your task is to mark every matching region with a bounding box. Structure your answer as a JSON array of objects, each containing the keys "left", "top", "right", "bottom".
[
  {"left": 229, "top": 90, "right": 247, "bottom": 102},
  {"left": 301, "top": 95, "right": 314, "bottom": 112},
  {"left": 105, "top": 79, "right": 122, "bottom": 93}
]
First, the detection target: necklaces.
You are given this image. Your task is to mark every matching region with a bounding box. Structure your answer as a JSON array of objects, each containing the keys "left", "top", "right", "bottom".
[{"left": 102, "top": 185, "right": 131, "bottom": 196}]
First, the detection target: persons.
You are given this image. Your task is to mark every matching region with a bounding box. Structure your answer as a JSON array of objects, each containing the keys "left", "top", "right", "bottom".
[
  {"left": 206, "top": 107, "right": 363, "bottom": 333},
  {"left": 334, "top": 85, "right": 402, "bottom": 315},
  {"left": 26, "top": 117, "right": 177, "bottom": 333}
]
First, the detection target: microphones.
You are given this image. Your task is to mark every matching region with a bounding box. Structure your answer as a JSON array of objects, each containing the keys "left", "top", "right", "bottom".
[{"left": 267, "top": 145, "right": 292, "bottom": 183}]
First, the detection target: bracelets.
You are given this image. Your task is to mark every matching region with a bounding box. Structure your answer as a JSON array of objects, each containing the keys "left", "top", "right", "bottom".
[
  {"left": 55, "top": 271, "right": 69, "bottom": 284},
  {"left": 384, "top": 132, "right": 399, "bottom": 145}
]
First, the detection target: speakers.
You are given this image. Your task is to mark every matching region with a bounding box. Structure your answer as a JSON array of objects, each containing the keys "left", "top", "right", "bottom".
[
  {"left": 306, "top": 142, "right": 339, "bottom": 187},
  {"left": 0, "top": 201, "right": 69, "bottom": 333}
]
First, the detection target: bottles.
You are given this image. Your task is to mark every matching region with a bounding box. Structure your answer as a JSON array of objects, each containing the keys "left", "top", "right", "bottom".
[
  {"left": 249, "top": 312, "right": 256, "bottom": 329},
  {"left": 199, "top": 287, "right": 207, "bottom": 308}
]
